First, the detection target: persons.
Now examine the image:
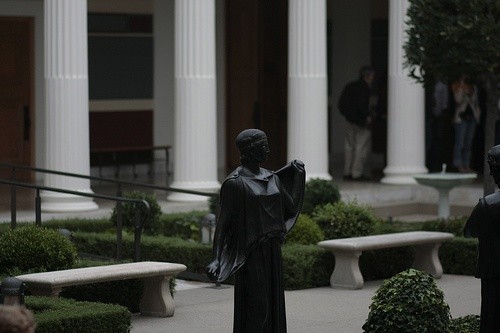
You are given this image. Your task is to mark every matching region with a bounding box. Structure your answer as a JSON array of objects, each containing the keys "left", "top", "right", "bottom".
[
  {"left": 205, "top": 130, "right": 306, "bottom": 333},
  {"left": 425, "top": 69, "right": 481, "bottom": 173},
  {"left": 338, "top": 66, "right": 374, "bottom": 180},
  {"left": 465, "top": 146, "right": 499, "bottom": 333}
]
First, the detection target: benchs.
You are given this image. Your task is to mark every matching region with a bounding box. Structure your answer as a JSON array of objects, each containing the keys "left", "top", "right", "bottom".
[
  {"left": 319, "top": 231, "right": 455, "bottom": 291},
  {"left": 16, "top": 261, "right": 186, "bottom": 317}
]
